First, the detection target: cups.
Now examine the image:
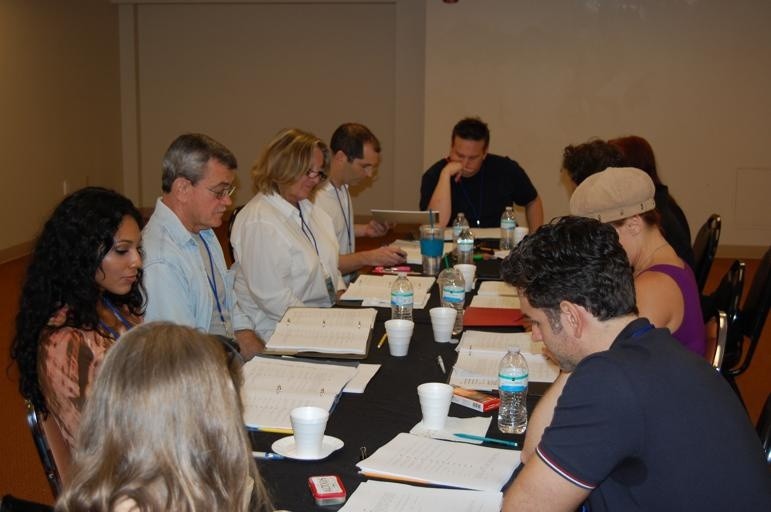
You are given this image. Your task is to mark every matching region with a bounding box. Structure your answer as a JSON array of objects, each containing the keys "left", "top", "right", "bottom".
[
  {"left": 515, "top": 227, "right": 528, "bottom": 246},
  {"left": 420, "top": 224, "right": 442, "bottom": 274},
  {"left": 428, "top": 264, "right": 477, "bottom": 345},
  {"left": 292, "top": 407, "right": 327, "bottom": 453},
  {"left": 417, "top": 382, "right": 454, "bottom": 431},
  {"left": 386, "top": 320, "right": 413, "bottom": 356}
]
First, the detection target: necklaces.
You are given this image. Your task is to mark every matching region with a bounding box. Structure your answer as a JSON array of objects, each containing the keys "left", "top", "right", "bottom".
[{"left": 634, "top": 243, "right": 670, "bottom": 275}]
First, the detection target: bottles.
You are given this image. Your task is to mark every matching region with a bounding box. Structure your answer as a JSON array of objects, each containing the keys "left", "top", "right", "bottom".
[
  {"left": 499, "top": 345, "right": 529, "bottom": 431},
  {"left": 453, "top": 212, "right": 474, "bottom": 264},
  {"left": 391, "top": 271, "right": 414, "bottom": 318},
  {"left": 501, "top": 208, "right": 515, "bottom": 249}
]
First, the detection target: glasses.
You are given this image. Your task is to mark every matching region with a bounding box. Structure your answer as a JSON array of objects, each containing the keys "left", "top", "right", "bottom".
[
  {"left": 206, "top": 185, "right": 239, "bottom": 199},
  {"left": 305, "top": 169, "right": 327, "bottom": 182}
]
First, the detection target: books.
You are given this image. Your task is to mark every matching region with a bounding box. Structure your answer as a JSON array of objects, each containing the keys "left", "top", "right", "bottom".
[
  {"left": 263, "top": 308, "right": 379, "bottom": 360},
  {"left": 384, "top": 238, "right": 460, "bottom": 267},
  {"left": 338, "top": 273, "right": 438, "bottom": 309},
  {"left": 447, "top": 329, "right": 562, "bottom": 394},
  {"left": 369, "top": 209, "right": 440, "bottom": 225},
  {"left": 338, "top": 476, "right": 505, "bottom": 512},
  {"left": 470, "top": 280, "right": 523, "bottom": 311},
  {"left": 237, "top": 354, "right": 358, "bottom": 434},
  {"left": 355, "top": 428, "right": 523, "bottom": 491},
  {"left": 436, "top": 226, "right": 511, "bottom": 242}
]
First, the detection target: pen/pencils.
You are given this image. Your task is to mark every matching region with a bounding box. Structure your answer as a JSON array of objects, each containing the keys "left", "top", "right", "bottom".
[
  {"left": 437, "top": 355, "right": 446, "bottom": 374},
  {"left": 251, "top": 451, "right": 285, "bottom": 460},
  {"left": 358, "top": 472, "right": 430, "bottom": 484},
  {"left": 480, "top": 247, "right": 498, "bottom": 251},
  {"left": 377, "top": 333, "right": 387, "bottom": 349},
  {"left": 454, "top": 434, "right": 518, "bottom": 447},
  {"left": 371, "top": 270, "right": 422, "bottom": 275}
]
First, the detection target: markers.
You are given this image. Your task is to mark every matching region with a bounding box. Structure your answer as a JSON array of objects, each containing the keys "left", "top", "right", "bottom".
[
  {"left": 374, "top": 266, "right": 412, "bottom": 272},
  {"left": 473, "top": 255, "right": 483, "bottom": 260}
]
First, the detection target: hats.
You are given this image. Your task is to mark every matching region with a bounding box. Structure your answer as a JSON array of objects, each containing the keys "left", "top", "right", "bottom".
[{"left": 566, "top": 165, "right": 657, "bottom": 224}]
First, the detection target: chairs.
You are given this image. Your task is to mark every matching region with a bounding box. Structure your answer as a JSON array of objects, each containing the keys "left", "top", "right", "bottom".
[
  {"left": 709, "top": 246, "right": 771, "bottom": 380},
  {"left": 750, "top": 389, "right": 770, "bottom": 465},
  {"left": 23, "top": 389, "right": 77, "bottom": 502},
  {"left": 693, "top": 258, "right": 741, "bottom": 318},
  {"left": 682, "top": 213, "right": 722, "bottom": 293}
]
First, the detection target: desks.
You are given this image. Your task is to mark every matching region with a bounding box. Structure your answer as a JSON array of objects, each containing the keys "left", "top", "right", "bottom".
[{"left": 243, "top": 220, "right": 562, "bottom": 511}]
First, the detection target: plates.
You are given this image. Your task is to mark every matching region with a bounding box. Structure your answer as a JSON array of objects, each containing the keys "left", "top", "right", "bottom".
[{"left": 272, "top": 433, "right": 344, "bottom": 461}]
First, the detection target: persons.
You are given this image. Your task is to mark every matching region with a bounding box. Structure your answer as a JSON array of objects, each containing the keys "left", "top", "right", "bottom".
[
  {"left": 420, "top": 117, "right": 545, "bottom": 234},
  {"left": 138, "top": 131, "right": 237, "bottom": 338},
  {"left": 598, "top": 137, "right": 695, "bottom": 263},
  {"left": 501, "top": 215, "right": 770, "bottom": 512},
  {"left": 6, "top": 185, "right": 144, "bottom": 459},
  {"left": 229, "top": 128, "right": 351, "bottom": 342},
  {"left": 568, "top": 164, "right": 705, "bottom": 359},
  {"left": 306, "top": 122, "right": 407, "bottom": 276},
  {"left": 52, "top": 318, "right": 287, "bottom": 512}
]
